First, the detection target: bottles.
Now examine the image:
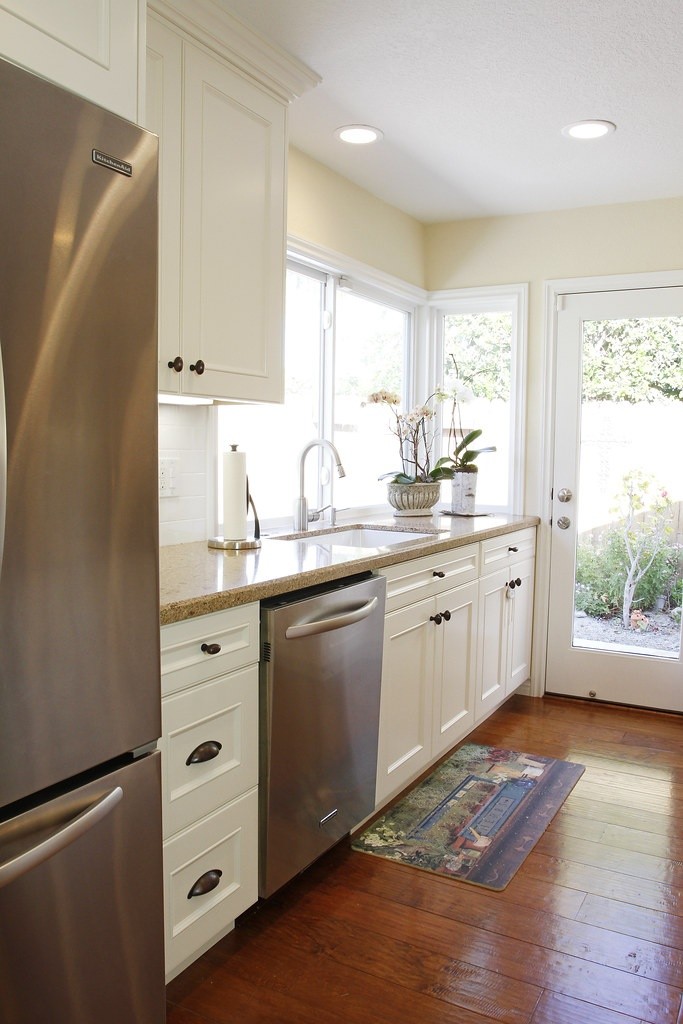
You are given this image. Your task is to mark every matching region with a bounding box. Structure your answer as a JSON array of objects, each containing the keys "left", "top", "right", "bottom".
[{"left": 222, "top": 444, "right": 247, "bottom": 541}]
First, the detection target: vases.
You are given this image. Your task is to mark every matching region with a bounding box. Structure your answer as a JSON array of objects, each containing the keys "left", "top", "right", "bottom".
[
  {"left": 387, "top": 483, "right": 441, "bottom": 517},
  {"left": 452, "top": 472, "right": 478, "bottom": 515}
]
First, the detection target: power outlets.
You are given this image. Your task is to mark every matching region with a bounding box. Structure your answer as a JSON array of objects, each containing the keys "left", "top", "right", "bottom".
[{"left": 158, "top": 458, "right": 172, "bottom": 498}]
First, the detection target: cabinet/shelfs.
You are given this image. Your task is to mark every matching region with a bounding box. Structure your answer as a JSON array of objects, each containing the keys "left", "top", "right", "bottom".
[
  {"left": 0, "top": 0, "right": 146, "bottom": 128},
  {"left": 146, "top": 6, "right": 288, "bottom": 406},
  {"left": 372, "top": 541, "right": 474, "bottom": 808},
  {"left": 474, "top": 526, "right": 537, "bottom": 724},
  {"left": 160, "top": 599, "right": 259, "bottom": 987}
]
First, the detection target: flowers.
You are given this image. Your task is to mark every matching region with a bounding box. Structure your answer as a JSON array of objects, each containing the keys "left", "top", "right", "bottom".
[
  {"left": 434, "top": 353, "right": 497, "bottom": 471},
  {"left": 361, "top": 389, "right": 455, "bottom": 484}
]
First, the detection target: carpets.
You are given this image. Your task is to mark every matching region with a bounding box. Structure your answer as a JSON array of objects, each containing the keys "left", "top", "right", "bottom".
[{"left": 351, "top": 741, "right": 587, "bottom": 892}]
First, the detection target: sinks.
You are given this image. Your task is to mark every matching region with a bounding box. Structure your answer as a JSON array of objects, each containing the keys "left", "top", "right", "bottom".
[{"left": 267, "top": 522, "right": 451, "bottom": 549}]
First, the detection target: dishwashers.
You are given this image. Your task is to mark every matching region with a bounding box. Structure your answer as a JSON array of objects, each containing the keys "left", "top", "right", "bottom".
[{"left": 256, "top": 572, "right": 390, "bottom": 902}]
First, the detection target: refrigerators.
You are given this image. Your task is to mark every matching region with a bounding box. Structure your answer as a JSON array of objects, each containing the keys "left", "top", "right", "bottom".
[{"left": 0, "top": 54, "right": 167, "bottom": 1024}]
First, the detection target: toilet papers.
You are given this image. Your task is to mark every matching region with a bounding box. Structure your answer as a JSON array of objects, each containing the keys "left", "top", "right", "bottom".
[{"left": 223, "top": 450, "right": 246, "bottom": 541}]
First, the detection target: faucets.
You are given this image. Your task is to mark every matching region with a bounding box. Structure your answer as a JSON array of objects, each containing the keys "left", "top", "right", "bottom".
[{"left": 299, "top": 440, "right": 345, "bottom": 531}]
